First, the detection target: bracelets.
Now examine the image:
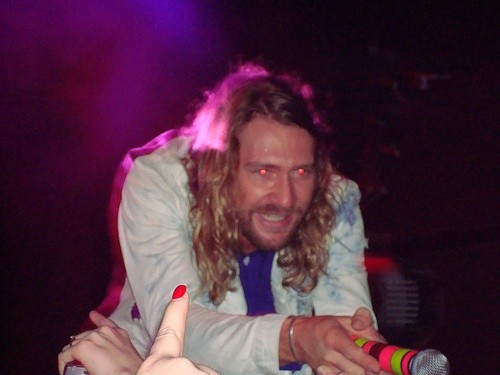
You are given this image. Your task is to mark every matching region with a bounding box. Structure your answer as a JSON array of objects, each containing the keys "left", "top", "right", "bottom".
[{"left": 288, "top": 314, "right": 310, "bottom": 365}]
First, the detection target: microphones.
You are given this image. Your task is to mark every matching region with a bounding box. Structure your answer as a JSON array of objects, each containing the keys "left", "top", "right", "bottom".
[{"left": 350, "top": 332, "right": 450, "bottom": 375}]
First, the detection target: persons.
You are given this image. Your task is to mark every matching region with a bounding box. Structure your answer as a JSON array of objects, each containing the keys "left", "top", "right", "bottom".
[
  {"left": 133, "top": 284, "right": 222, "bottom": 375},
  {"left": 58, "top": 284, "right": 144, "bottom": 375},
  {"left": 78, "top": 59, "right": 398, "bottom": 375}
]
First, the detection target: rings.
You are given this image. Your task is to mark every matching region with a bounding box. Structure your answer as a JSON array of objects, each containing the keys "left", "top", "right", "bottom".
[{"left": 68, "top": 335, "right": 77, "bottom": 348}]
